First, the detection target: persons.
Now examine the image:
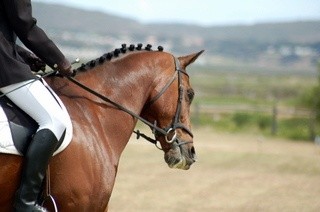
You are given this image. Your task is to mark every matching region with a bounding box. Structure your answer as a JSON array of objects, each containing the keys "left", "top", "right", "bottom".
[{"left": 0, "top": 0, "right": 73, "bottom": 212}]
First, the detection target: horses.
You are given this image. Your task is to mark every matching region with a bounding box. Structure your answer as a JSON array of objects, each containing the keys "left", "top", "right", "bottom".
[{"left": 0, "top": 43, "right": 204, "bottom": 212}]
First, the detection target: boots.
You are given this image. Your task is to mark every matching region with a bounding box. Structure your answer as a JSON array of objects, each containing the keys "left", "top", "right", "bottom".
[{"left": 11, "top": 128, "right": 60, "bottom": 212}]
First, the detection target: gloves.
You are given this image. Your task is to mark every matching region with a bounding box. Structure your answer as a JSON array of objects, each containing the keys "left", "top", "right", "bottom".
[
  {"left": 27, "top": 53, "right": 46, "bottom": 74},
  {"left": 60, "top": 63, "right": 75, "bottom": 77}
]
